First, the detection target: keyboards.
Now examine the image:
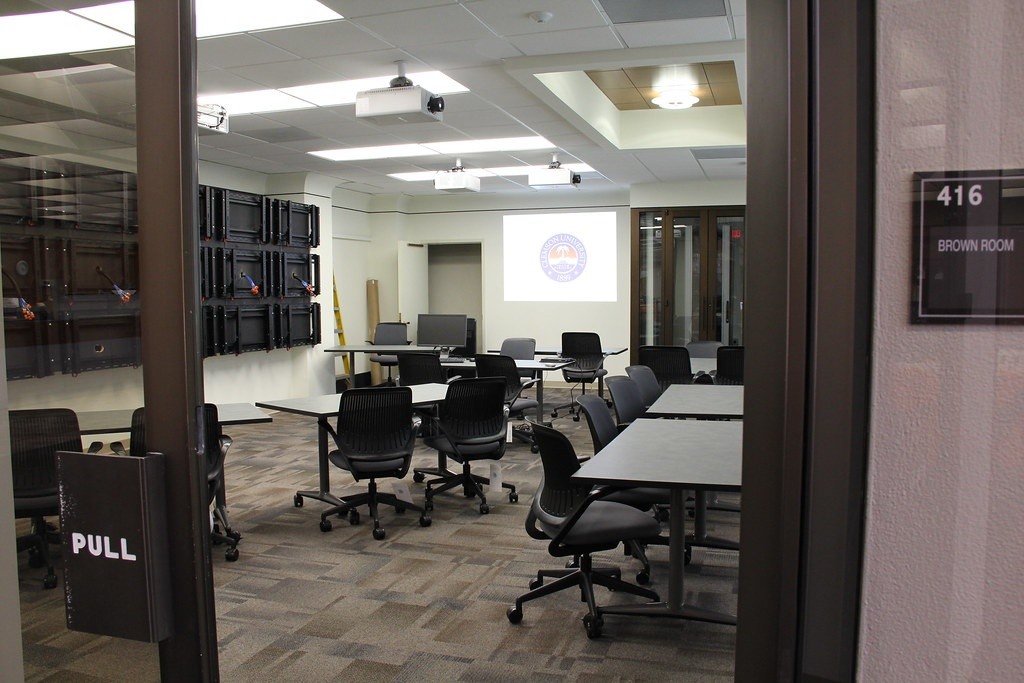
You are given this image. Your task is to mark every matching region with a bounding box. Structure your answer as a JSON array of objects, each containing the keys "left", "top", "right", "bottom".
[{"left": 439, "top": 358, "right": 466, "bottom": 363}]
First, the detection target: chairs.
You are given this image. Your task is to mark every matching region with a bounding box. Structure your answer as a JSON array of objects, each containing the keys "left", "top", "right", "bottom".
[
  {"left": 318, "top": 312, "right": 743, "bottom": 641},
  {"left": 8, "top": 408, "right": 104, "bottom": 588},
  {"left": 110, "top": 404, "right": 242, "bottom": 560}
]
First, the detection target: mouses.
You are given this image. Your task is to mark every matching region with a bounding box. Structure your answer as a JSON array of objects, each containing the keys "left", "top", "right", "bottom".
[{"left": 470, "top": 358, "right": 475, "bottom": 362}]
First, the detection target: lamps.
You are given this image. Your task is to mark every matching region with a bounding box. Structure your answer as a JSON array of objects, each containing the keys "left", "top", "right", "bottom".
[{"left": 651, "top": 90, "right": 699, "bottom": 108}]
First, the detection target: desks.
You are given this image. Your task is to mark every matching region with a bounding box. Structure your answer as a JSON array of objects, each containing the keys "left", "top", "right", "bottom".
[
  {"left": 440, "top": 359, "right": 575, "bottom": 453},
  {"left": 324, "top": 346, "right": 442, "bottom": 390},
  {"left": 77, "top": 403, "right": 273, "bottom": 543},
  {"left": 256, "top": 383, "right": 451, "bottom": 523},
  {"left": 645, "top": 383, "right": 743, "bottom": 522},
  {"left": 569, "top": 418, "right": 743, "bottom": 628},
  {"left": 487, "top": 347, "right": 628, "bottom": 409}
]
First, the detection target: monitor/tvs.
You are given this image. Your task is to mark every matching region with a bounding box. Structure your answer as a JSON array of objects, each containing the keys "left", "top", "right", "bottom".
[{"left": 418, "top": 315, "right": 467, "bottom": 357}]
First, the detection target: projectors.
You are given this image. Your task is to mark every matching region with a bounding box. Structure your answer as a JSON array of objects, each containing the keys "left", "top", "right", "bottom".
[
  {"left": 197, "top": 106, "right": 229, "bottom": 136},
  {"left": 355, "top": 85, "right": 444, "bottom": 127},
  {"left": 433, "top": 174, "right": 481, "bottom": 193},
  {"left": 528, "top": 168, "right": 581, "bottom": 190}
]
